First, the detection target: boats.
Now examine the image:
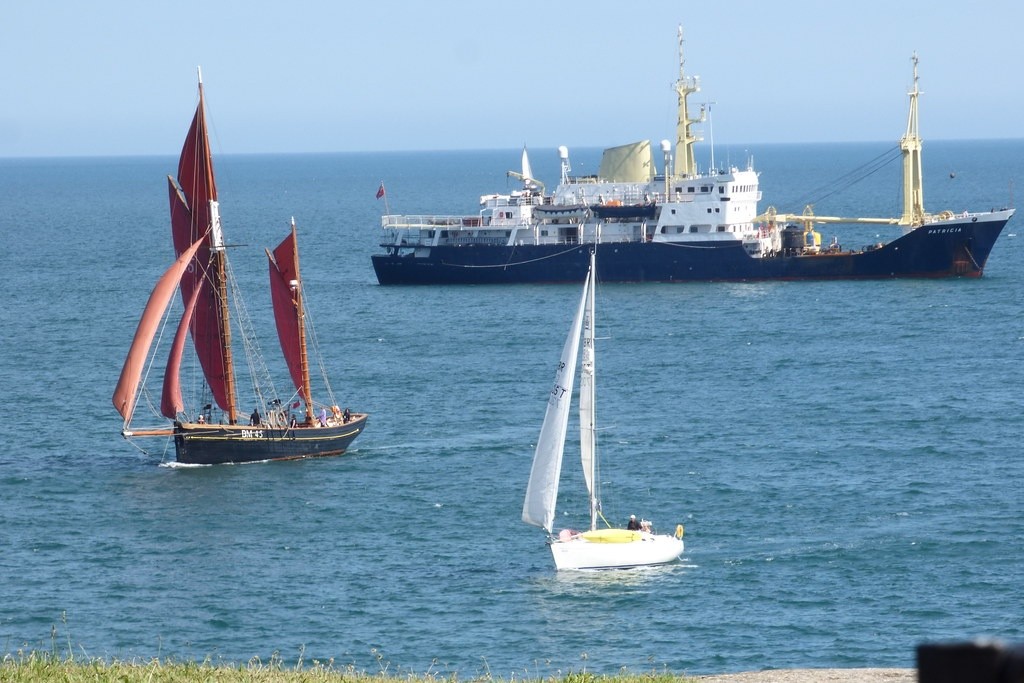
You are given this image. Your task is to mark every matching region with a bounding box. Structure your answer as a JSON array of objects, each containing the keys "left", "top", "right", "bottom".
[
  {"left": 588, "top": 198, "right": 658, "bottom": 218},
  {"left": 370, "top": 19, "right": 1015, "bottom": 286},
  {"left": 534, "top": 205, "right": 587, "bottom": 218}
]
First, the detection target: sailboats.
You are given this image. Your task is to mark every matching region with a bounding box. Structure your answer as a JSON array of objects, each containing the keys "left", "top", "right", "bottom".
[
  {"left": 109, "top": 65, "right": 371, "bottom": 470},
  {"left": 519, "top": 225, "right": 686, "bottom": 573}
]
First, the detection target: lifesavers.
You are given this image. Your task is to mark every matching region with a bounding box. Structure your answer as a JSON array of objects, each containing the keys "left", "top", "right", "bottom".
[
  {"left": 334, "top": 406, "right": 341, "bottom": 419},
  {"left": 677, "top": 524, "right": 683, "bottom": 538}
]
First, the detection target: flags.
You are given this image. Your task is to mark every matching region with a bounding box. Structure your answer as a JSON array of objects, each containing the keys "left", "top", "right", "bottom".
[
  {"left": 293, "top": 401, "right": 300, "bottom": 409},
  {"left": 377, "top": 184, "right": 385, "bottom": 199}
]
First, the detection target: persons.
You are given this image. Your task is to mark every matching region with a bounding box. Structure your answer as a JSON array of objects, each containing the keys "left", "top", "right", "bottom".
[
  {"left": 316, "top": 406, "right": 326, "bottom": 427},
  {"left": 197, "top": 415, "right": 207, "bottom": 425},
  {"left": 250, "top": 408, "right": 262, "bottom": 426},
  {"left": 291, "top": 414, "right": 296, "bottom": 428},
  {"left": 628, "top": 515, "right": 643, "bottom": 530},
  {"left": 343, "top": 408, "right": 350, "bottom": 424}
]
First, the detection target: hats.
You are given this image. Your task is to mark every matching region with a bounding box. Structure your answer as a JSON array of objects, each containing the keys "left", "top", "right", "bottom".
[
  {"left": 291, "top": 414, "right": 297, "bottom": 418},
  {"left": 630, "top": 515, "right": 636, "bottom": 519}
]
